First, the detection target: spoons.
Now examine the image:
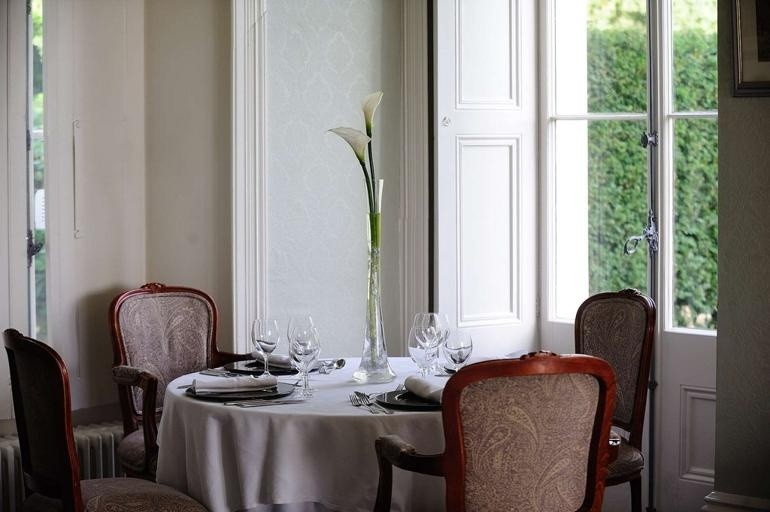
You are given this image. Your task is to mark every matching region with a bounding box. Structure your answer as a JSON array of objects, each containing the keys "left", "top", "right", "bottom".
[{"left": 333, "top": 359, "right": 345, "bottom": 369}]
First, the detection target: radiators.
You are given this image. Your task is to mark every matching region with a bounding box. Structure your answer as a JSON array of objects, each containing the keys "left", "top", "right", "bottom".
[{"left": 2, "top": 415, "right": 126, "bottom": 512}]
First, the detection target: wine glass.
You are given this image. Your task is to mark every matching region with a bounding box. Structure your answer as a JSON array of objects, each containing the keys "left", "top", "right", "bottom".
[
  {"left": 286, "top": 316, "right": 320, "bottom": 400},
  {"left": 252, "top": 319, "right": 280, "bottom": 375},
  {"left": 407, "top": 313, "right": 474, "bottom": 380}
]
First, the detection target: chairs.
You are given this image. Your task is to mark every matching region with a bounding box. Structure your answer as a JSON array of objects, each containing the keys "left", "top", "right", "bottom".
[
  {"left": 369, "top": 352, "right": 618, "bottom": 512},
  {"left": 521, "top": 287, "right": 655, "bottom": 509},
  {"left": 0, "top": 329, "right": 210, "bottom": 512},
  {"left": 108, "top": 284, "right": 257, "bottom": 482}
]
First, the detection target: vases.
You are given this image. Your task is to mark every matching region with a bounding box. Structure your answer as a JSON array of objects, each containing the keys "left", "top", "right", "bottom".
[{"left": 351, "top": 209, "right": 398, "bottom": 385}]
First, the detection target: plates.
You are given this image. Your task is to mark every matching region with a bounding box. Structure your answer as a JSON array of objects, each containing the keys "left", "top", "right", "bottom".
[
  {"left": 187, "top": 382, "right": 294, "bottom": 402},
  {"left": 224, "top": 360, "right": 301, "bottom": 375},
  {"left": 375, "top": 390, "right": 445, "bottom": 411}
]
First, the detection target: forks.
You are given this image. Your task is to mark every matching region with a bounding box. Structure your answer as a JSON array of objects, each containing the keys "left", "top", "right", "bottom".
[{"left": 349, "top": 391, "right": 390, "bottom": 415}]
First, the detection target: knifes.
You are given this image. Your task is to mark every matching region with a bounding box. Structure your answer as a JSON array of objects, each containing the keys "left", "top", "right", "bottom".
[{"left": 226, "top": 398, "right": 302, "bottom": 407}]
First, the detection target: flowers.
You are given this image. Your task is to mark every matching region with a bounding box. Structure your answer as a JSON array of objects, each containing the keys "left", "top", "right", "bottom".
[{"left": 322, "top": 89, "right": 385, "bottom": 365}]
